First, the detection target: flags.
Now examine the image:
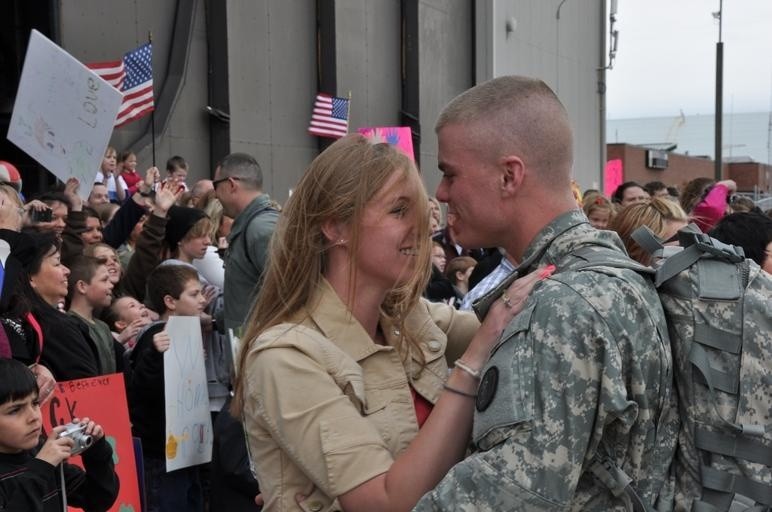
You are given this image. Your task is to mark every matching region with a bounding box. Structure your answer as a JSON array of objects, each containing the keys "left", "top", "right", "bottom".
[
  {"left": 308, "top": 94, "right": 349, "bottom": 138},
  {"left": 82, "top": 45, "right": 155, "bottom": 129},
  {"left": 712, "top": 11, "right": 720, "bottom": 18}
]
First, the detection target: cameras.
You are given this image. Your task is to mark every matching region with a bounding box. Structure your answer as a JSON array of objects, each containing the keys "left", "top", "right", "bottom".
[
  {"left": 701, "top": 184, "right": 737, "bottom": 206},
  {"left": 57, "top": 422, "right": 93, "bottom": 457},
  {"left": 34, "top": 209, "right": 52, "bottom": 222}
]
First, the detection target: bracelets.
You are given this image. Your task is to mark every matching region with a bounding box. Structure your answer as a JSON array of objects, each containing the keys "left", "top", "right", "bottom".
[
  {"left": 454, "top": 359, "right": 481, "bottom": 379},
  {"left": 443, "top": 383, "right": 478, "bottom": 397}
]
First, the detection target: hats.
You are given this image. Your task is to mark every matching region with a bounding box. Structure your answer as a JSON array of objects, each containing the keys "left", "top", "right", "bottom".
[{"left": 165, "top": 206, "right": 210, "bottom": 242}]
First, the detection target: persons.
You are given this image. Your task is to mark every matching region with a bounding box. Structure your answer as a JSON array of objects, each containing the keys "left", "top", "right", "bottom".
[
  {"left": 411, "top": 74, "right": 678, "bottom": 512},
  {"left": 226, "top": 133, "right": 557, "bottom": 512},
  {"left": 0, "top": 145, "right": 284, "bottom": 511},
  {"left": 420, "top": 196, "right": 519, "bottom": 311},
  {"left": 571, "top": 177, "right": 772, "bottom": 275}
]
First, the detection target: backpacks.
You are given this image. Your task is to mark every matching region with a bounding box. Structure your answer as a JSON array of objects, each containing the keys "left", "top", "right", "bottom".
[{"left": 552, "top": 222, "right": 772, "bottom": 512}]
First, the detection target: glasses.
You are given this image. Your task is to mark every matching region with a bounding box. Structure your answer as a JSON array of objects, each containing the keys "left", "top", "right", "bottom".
[{"left": 213, "top": 177, "right": 240, "bottom": 190}]
